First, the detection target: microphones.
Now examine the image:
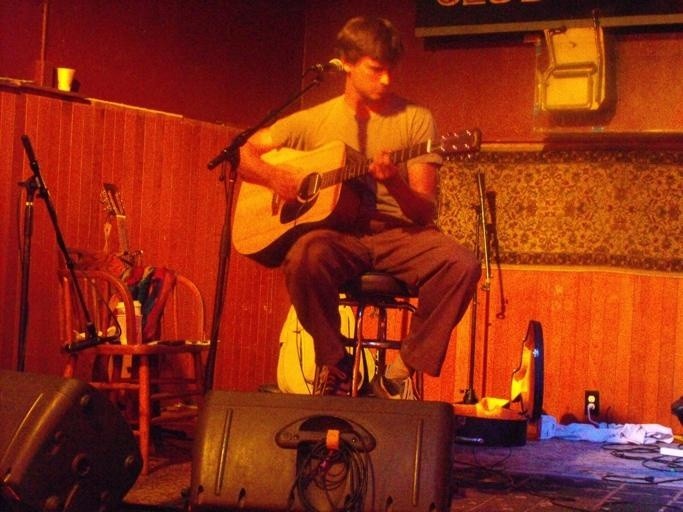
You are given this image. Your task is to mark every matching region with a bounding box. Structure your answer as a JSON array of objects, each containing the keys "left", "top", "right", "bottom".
[
  {"left": 308, "top": 59, "right": 346, "bottom": 75},
  {"left": 63, "top": 335, "right": 117, "bottom": 355}
]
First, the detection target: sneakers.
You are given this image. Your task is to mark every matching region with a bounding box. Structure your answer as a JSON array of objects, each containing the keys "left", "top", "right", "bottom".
[
  {"left": 315, "top": 353, "right": 356, "bottom": 399},
  {"left": 372, "top": 365, "right": 421, "bottom": 400}
]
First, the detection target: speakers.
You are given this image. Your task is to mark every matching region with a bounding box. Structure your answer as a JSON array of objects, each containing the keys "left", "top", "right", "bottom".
[
  {"left": 0, "top": 369, "right": 144, "bottom": 512},
  {"left": 186, "top": 390, "right": 454, "bottom": 512}
]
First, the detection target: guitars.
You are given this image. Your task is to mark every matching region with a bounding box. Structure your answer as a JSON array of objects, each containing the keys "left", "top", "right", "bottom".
[
  {"left": 97, "top": 183, "right": 164, "bottom": 388},
  {"left": 233, "top": 128, "right": 481, "bottom": 267}
]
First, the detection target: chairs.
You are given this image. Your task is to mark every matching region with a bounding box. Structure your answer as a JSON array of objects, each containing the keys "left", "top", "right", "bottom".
[{"left": 56, "top": 247, "right": 209, "bottom": 477}]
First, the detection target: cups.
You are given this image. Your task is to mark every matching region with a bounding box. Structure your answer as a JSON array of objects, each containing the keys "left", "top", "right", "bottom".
[{"left": 57, "top": 67, "right": 75, "bottom": 91}]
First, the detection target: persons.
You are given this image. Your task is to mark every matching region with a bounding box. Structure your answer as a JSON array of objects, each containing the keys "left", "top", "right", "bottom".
[{"left": 237, "top": 16, "right": 481, "bottom": 400}]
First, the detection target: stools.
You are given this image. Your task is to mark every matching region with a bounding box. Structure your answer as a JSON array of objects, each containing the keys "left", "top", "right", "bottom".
[{"left": 339, "top": 272, "right": 424, "bottom": 402}]
